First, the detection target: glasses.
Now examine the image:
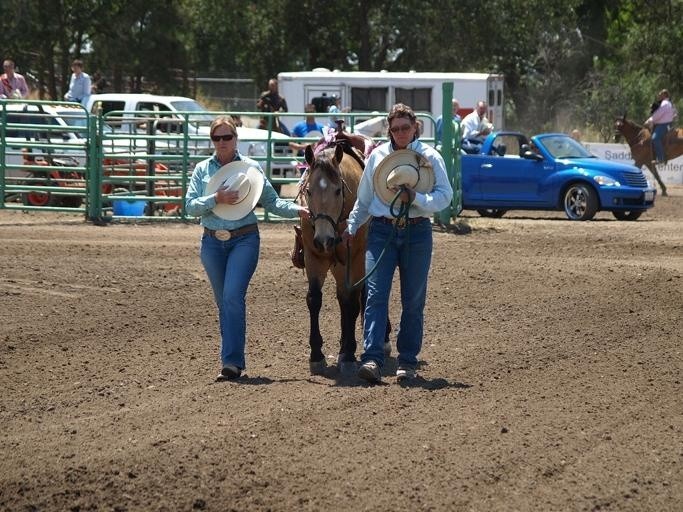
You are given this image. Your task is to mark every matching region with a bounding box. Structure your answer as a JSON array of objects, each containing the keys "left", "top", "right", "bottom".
[
  {"left": 211, "top": 134, "right": 233, "bottom": 142},
  {"left": 388, "top": 123, "right": 413, "bottom": 135}
]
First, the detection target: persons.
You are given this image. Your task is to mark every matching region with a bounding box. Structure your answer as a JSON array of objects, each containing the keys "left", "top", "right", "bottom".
[
  {"left": 291, "top": 103, "right": 325, "bottom": 204},
  {"left": 184, "top": 115, "right": 312, "bottom": 382},
  {"left": 256, "top": 77, "right": 288, "bottom": 131},
  {"left": 570, "top": 130, "right": 581, "bottom": 143},
  {"left": 341, "top": 104, "right": 454, "bottom": 382},
  {"left": 63, "top": 59, "right": 90, "bottom": 106},
  {"left": 459, "top": 100, "right": 493, "bottom": 154},
  {"left": 643, "top": 89, "right": 674, "bottom": 169},
  {"left": 0, "top": 59, "right": 28, "bottom": 100},
  {"left": 327, "top": 92, "right": 351, "bottom": 130},
  {"left": 529, "top": 139, "right": 539, "bottom": 155},
  {"left": 434, "top": 99, "right": 462, "bottom": 142}
]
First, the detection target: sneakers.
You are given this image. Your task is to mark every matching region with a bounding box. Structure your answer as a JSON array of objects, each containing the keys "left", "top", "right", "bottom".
[
  {"left": 221, "top": 366, "right": 241, "bottom": 378},
  {"left": 396, "top": 366, "right": 415, "bottom": 382},
  {"left": 216, "top": 374, "right": 228, "bottom": 381},
  {"left": 358, "top": 361, "right": 381, "bottom": 385}
]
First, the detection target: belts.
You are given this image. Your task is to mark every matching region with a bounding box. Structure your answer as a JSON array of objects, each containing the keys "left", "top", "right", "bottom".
[
  {"left": 204, "top": 224, "right": 258, "bottom": 241},
  {"left": 374, "top": 216, "right": 423, "bottom": 228}
]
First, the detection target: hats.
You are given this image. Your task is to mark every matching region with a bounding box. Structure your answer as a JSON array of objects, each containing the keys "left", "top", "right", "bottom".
[
  {"left": 373, "top": 149, "right": 434, "bottom": 209},
  {"left": 204, "top": 161, "right": 264, "bottom": 221}
]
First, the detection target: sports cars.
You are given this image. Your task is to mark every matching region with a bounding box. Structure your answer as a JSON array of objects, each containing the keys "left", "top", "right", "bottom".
[
  {"left": 462, "top": 130, "right": 656, "bottom": 221},
  {"left": 100, "top": 204, "right": 101, "bottom": 207}
]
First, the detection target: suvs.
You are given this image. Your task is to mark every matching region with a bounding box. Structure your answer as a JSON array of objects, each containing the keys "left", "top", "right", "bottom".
[
  {"left": 0, "top": 100, "right": 188, "bottom": 188},
  {"left": 22, "top": 144, "right": 181, "bottom": 213}
]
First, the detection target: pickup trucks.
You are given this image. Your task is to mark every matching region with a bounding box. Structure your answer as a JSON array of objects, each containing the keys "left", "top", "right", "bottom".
[{"left": 83, "top": 92, "right": 295, "bottom": 202}]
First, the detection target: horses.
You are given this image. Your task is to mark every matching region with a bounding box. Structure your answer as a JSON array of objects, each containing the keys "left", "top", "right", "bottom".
[
  {"left": 610, "top": 108, "right": 683, "bottom": 199},
  {"left": 290, "top": 128, "right": 396, "bottom": 380}
]
